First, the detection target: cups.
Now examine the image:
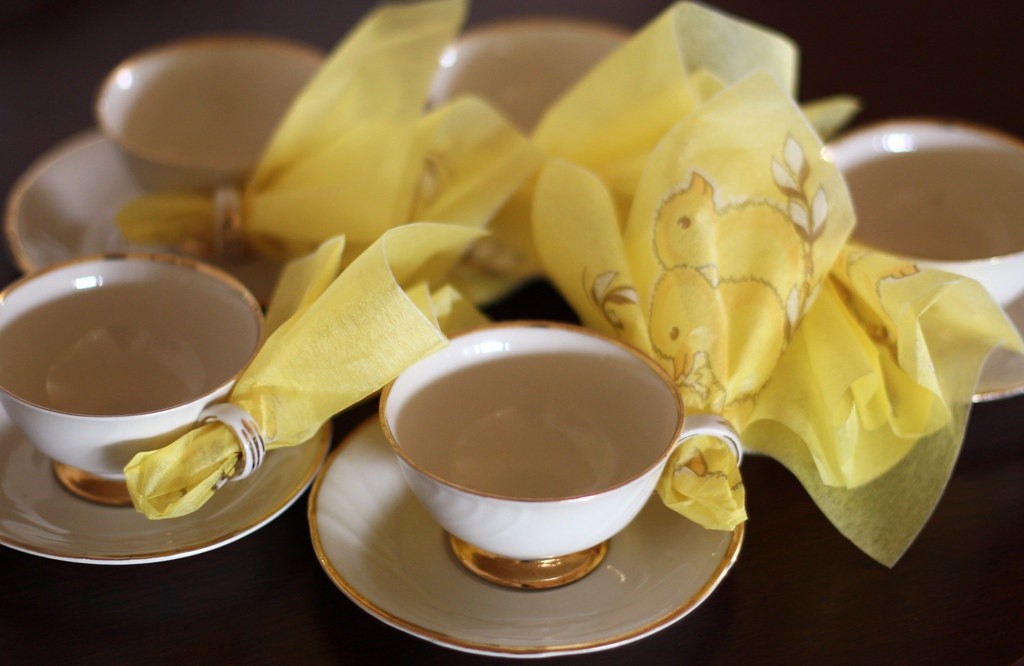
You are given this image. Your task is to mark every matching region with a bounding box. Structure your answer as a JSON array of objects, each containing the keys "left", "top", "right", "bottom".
[
  {"left": 95, "top": 32, "right": 336, "bottom": 215},
  {"left": 1, "top": 253, "right": 266, "bottom": 507},
  {"left": 378, "top": 325, "right": 684, "bottom": 592},
  {"left": 415, "top": 20, "right": 657, "bottom": 177},
  {"left": 815, "top": 116, "right": 1023, "bottom": 330}
]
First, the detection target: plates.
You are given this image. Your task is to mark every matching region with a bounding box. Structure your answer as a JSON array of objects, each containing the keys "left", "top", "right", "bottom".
[
  {"left": 307, "top": 413, "right": 745, "bottom": 659},
  {"left": 0, "top": 385, "right": 335, "bottom": 564},
  {"left": 905, "top": 275, "right": 1023, "bottom": 405},
  {"left": 4, "top": 118, "right": 194, "bottom": 283}
]
